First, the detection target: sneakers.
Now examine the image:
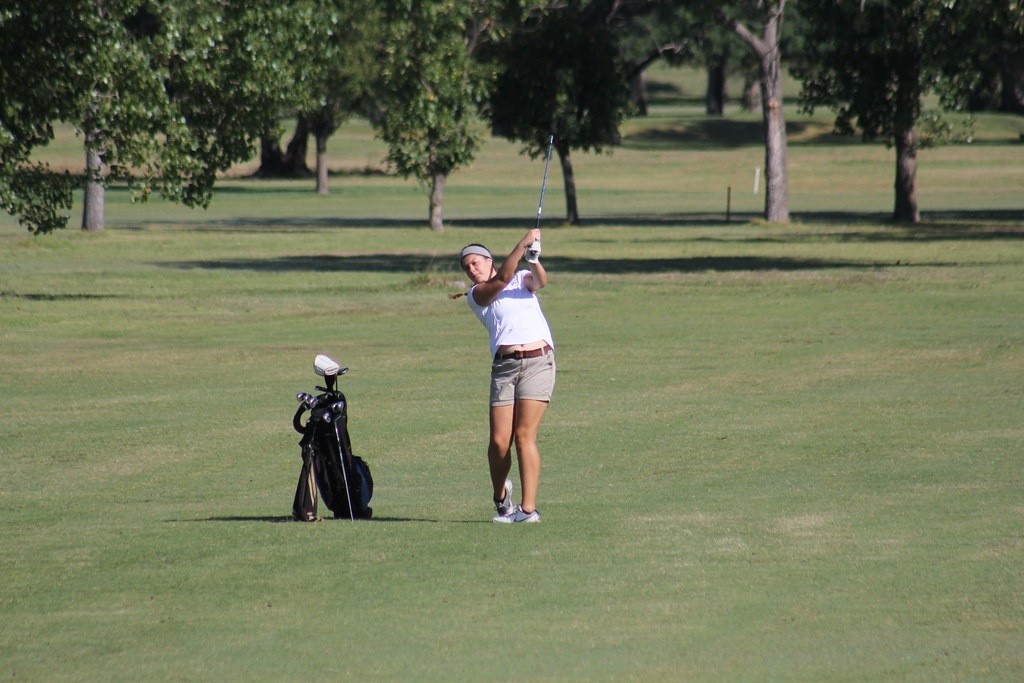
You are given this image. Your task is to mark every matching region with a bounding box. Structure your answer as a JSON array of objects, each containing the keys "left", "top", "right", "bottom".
[
  {"left": 493, "top": 479, "right": 514, "bottom": 517},
  {"left": 492, "top": 504, "right": 541, "bottom": 524}
]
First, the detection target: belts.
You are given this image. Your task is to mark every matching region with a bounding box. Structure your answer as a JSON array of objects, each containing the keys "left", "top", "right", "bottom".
[{"left": 495, "top": 344, "right": 551, "bottom": 359}]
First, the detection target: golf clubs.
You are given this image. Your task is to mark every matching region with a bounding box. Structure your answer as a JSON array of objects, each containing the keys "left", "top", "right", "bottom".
[
  {"left": 297, "top": 366, "right": 350, "bottom": 423},
  {"left": 529, "top": 135, "right": 554, "bottom": 255}
]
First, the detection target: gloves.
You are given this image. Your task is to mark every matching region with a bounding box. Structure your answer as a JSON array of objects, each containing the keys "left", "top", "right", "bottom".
[{"left": 523, "top": 239, "right": 541, "bottom": 264}]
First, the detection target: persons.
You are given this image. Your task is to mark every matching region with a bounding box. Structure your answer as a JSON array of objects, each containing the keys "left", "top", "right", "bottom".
[{"left": 446, "top": 228, "right": 557, "bottom": 525}]
